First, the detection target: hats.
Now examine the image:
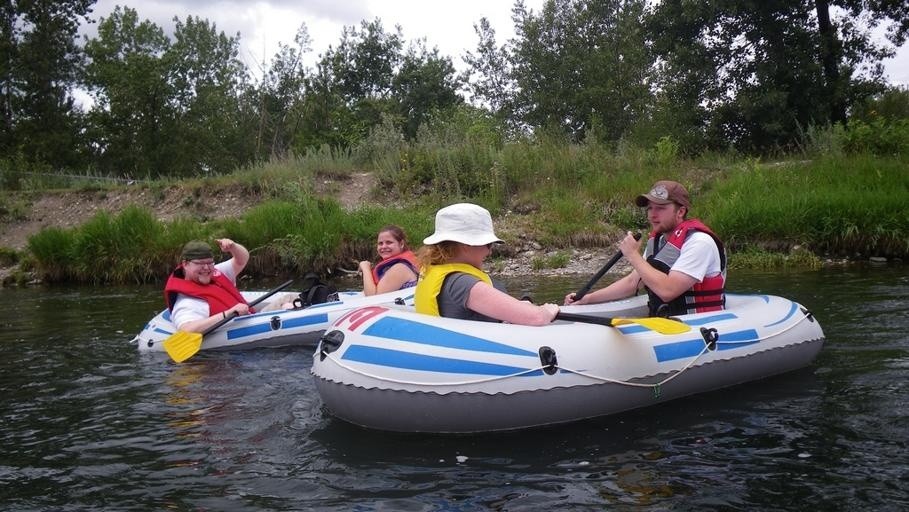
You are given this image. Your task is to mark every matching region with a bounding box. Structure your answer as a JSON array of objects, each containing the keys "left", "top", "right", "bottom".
[
  {"left": 181, "top": 239, "right": 213, "bottom": 259},
  {"left": 635, "top": 181, "right": 689, "bottom": 210},
  {"left": 423, "top": 202, "right": 506, "bottom": 247}
]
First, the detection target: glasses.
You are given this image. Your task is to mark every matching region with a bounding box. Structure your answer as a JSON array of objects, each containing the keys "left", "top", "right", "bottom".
[{"left": 190, "top": 261, "right": 215, "bottom": 266}]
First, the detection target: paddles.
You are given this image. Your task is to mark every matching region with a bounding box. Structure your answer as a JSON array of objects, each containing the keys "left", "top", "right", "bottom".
[
  {"left": 161, "top": 277, "right": 295, "bottom": 364},
  {"left": 555, "top": 312, "right": 691, "bottom": 335}
]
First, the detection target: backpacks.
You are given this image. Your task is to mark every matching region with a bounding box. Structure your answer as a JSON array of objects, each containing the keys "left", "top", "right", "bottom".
[{"left": 299, "top": 284, "right": 338, "bottom": 306}]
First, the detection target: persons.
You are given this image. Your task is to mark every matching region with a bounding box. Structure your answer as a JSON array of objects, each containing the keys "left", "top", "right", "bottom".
[
  {"left": 166, "top": 237, "right": 256, "bottom": 332},
  {"left": 415, "top": 204, "right": 560, "bottom": 326},
  {"left": 359, "top": 226, "right": 420, "bottom": 296},
  {"left": 564, "top": 180, "right": 727, "bottom": 317}
]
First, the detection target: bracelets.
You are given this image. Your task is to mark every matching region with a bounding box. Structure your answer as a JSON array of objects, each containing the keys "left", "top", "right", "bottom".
[{"left": 222, "top": 311, "right": 226, "bottom": 319}]
